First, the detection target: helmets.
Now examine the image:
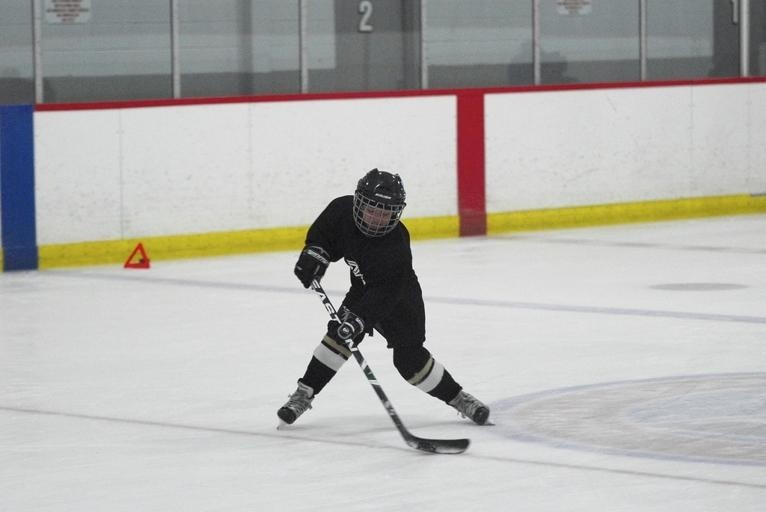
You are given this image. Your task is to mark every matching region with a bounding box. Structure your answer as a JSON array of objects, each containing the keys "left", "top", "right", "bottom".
[{"left": 352, "top": 168, "right": 406, "bottom": 239}]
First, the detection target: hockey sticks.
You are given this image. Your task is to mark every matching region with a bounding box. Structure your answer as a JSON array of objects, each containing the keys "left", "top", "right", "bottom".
[{"left": 312, "top": 277, "right": 470, "bottom": 455}]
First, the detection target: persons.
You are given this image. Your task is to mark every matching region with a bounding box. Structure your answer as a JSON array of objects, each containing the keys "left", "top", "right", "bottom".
[{"left": 276, "top": 167, "right": 489, "bottom": 427}]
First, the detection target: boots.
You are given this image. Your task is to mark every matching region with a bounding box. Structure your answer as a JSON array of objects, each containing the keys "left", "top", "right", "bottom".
[
  {"left": 450, "top": 391, "right": 489, "bottom": 426},
  {"left": 278, "top": 377, "right": 315, "bottom": 424}
]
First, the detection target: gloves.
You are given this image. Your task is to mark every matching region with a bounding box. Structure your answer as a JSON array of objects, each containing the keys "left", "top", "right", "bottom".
[
  {"left": 294, "top": 242, "right": 329, "bottom": 289},
  {"left": 328, "top": 308, "right": 366, "bottom": 345}
]
